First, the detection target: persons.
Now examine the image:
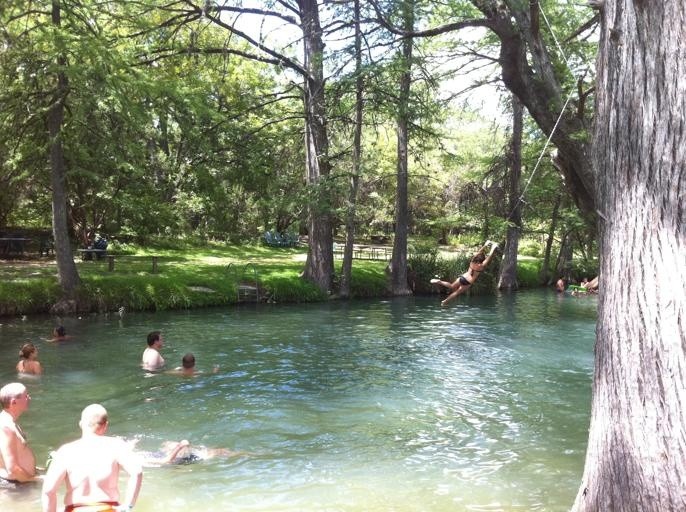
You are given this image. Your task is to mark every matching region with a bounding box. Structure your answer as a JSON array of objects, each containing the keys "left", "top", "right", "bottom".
[
  {"left": 143, "top": 440, "right": 241, "bottom": 468},
  {"left": 141, "top": 331, "right": 165, "bottom": 371},
  {"left": 0, "top": 382, "right": 46, "bottom": 489},
  {"left": 571, "top": 288, "right": 580, "bottom": 296},
  {"left": 15, "top": 342, "right": 42, "bottom": 378},
  {"left": 172, "top": 353, "right": 219, "bottom": 376},
  {"left": 556, "top": 276, "right": 565, "bottom": 294},
  {"left": 430, "top": 240, "right": 499, "bottom": 307},
  {"left": 39, "top": 403, "right": 142, "bottom": 511},
  {"left": 38, "top": 325, "right": 73, "bottom": 343},
  {"left": 581, "top": 277, "right": 591, "bottom": 289}
]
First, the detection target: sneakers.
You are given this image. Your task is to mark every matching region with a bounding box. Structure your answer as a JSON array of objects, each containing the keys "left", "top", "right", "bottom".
[
  {"left": 441, "top": 299, "right": 448, "bottom": 306},
  {"left": 431, "top": 279, "right": 440, "bottom": 283}
]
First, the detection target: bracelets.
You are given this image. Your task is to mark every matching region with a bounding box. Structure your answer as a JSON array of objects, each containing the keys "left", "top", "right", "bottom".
[
  {"left": 175, "top": 444, "right": 184, "bottom": 449},
  {"left": 123, "top": 501, "right": 134, "bottom": 509}
]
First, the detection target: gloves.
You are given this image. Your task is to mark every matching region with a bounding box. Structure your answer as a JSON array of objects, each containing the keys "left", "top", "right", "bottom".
[
  {"left": 492, "top": 244, "right": 498, "bottom": 250},
  {"left": 485, "top": 240, "right": 489, "bottom": 246}
]
